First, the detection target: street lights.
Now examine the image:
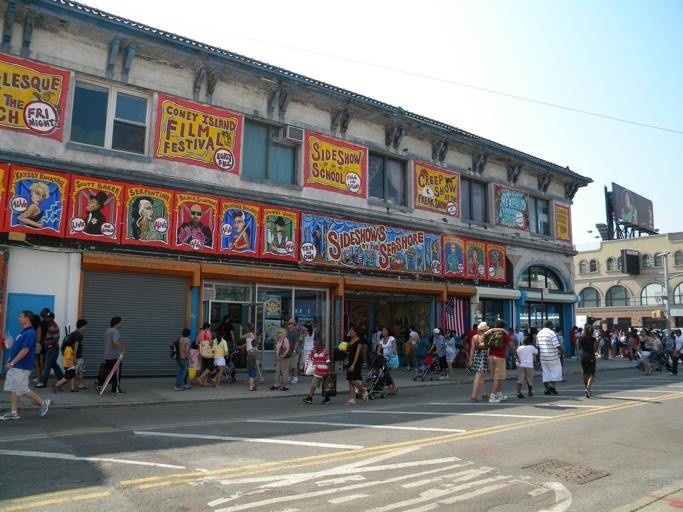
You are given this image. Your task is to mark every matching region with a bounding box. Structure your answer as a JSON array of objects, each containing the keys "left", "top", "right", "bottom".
[{"left": 657, "top": 250, "right": 671, "bottom": 332}]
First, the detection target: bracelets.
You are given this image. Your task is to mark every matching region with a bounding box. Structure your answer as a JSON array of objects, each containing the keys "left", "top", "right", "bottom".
[{"left": 10, "top": 361, "right": 14, "bottom": 367}]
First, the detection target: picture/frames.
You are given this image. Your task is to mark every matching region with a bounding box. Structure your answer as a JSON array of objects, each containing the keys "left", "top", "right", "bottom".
[
  {"left": 228, "top": 304, "right": 242, "bottom": 323},
  {"left": 211, "top": 303, "right": 221, "bottom": 322}
]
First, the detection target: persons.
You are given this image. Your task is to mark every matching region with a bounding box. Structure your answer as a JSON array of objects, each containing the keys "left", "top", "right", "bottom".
[
  {"left": 302, "top": 323, "right": 468, "bottom": 406},
  {"left": 592, "top": 326, "right": 683, "bottom": 375},
  {"left": 0, "top": 311, "right": 51, "bottom": 421},
  {"left": 31, "top": 307, "right": 89, "bottom": 392},
  {"left": 620, "top": 190, "right": 636, "bottom": 223},
  {"left": 465, "top": 320, "right": 598, "bottom": 403},
  {"left": 173, "top": 318, "right": 315, "bottom": 391},
  {"left": 96, "top": 317, "right": 127, "bottom": 395}
]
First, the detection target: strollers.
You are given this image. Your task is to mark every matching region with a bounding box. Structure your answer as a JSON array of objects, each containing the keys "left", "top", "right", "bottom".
[
  {"left": 206, "top": 347, "right": 240, "bottom": 384},
  {"left": 411, "top": 354, "right": 437, "bottom": 383},
  {"left": 355, "top": 354, "right": 394, "bottom": 400}
]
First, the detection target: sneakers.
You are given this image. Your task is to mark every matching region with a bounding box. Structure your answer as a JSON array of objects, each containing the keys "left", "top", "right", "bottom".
[
  {"left": 174, "top": 377, "right": 224, "bottom": 391},
  {"left": 436, "top": 374, "right": 455, "bottom": 381},
  {"left": 322, "top": 398, "right": 331, "bottom": 404},
  {"left": 516, "top": 385, "right": 557, "bottom": 398},
  {"left": 38, "top": 399, "right": 50, "bottom": 417},
  {"left": 52, "top": 383, "right": 89, "bottom": 392},
  {"left": 386, "top": 387, "right": 398, "bottom": 395},
  {"left": 246, "top": 376, "right": 299, "bottom": 391},
  {"left": 344, "top": 399, "right": 356, "bottom": 406},
  {"left": 362, "top": 391, "right": 368, "bottom": 404},
  {"left": 0, "top": 411, "right": 21, "bottom": 420},
  {"left": 584, "top": 389, "right": 591, "bottom": 399},
  {"left": 94, "top": 379, "right": 101, "bottom": 393},
  {"left": 471, "top": 392, "right": 508, "bottom": 403},
  {"left": 112, "top": 389, "right": 126, "bottom": 395},
  {"left": 302, "top": 398, "right": 312, "bottom": 404},
  {"left": 32, "top": 377, "right": 47, "bottom": 388}
]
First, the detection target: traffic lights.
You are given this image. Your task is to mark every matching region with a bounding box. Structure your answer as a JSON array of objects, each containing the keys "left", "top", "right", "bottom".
[{"left": 656, "top": 310, "right": 667, "bottom": 319}]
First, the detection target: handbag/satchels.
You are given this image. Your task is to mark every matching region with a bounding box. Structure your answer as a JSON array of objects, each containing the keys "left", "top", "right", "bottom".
[
  {"left": 98, "top": 363, "right": 112, "bottom": 385},
  {"left": 645, "top": 343, "right": 654, "bottom": 350},
  {"left": 251, "top": 338, "right": 259, "bottom": 348},
  {"left": 199, "top": 340, "right": 215, "bottom": 358},
  {"left": 387, "top": 346, "right": 400, "bottom": 369},
  {"left": 593, "top": 341, "right": 600, "bottom": 353},
  {"left": 187, "top": 368, "right": 196, "bottom": 379},
  {"left": 279, "top": 346, "right": 292, "bottom": 358},
  {"left": 673, "top": 351, "right": 681, "bottom": 359},
  {"left": 322, "top": 373, "right": 337, "bottom": 397}
]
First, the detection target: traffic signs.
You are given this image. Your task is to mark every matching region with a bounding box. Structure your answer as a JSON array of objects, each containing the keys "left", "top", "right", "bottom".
[{"left": 659, "top": 296, "right": 668, "bottom": 300}]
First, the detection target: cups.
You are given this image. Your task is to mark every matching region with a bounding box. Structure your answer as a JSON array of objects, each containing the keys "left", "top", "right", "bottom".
[{"left": 3, "top": 336, "right": 13, "bottom": 349}]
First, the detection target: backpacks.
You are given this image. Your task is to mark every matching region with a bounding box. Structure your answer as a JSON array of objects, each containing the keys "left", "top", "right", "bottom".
[
  {"left": 487, "top": 331, "right": 506, "bottom": 350},
  {"left": 170, "top": 336, "right": 182, "bottom": 360},
  {"left": 62, "top": 335, "right": 72, "bottom": 355}
]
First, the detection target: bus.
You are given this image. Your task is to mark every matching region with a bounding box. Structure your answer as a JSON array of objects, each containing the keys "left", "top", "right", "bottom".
[{"left": 518, "top": 315, "right": 596, "bottom": 344}]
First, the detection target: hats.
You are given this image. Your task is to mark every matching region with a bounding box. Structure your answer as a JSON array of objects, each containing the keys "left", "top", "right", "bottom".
[
  {"left": 433, "top": 328, "right": 440, "bottom": 334},
  {"left": 477, "top": 322, "right": 490, "bottom": 331},
  {"left": 312, "top": 340, "right": 325, "bottom": 349}
]
